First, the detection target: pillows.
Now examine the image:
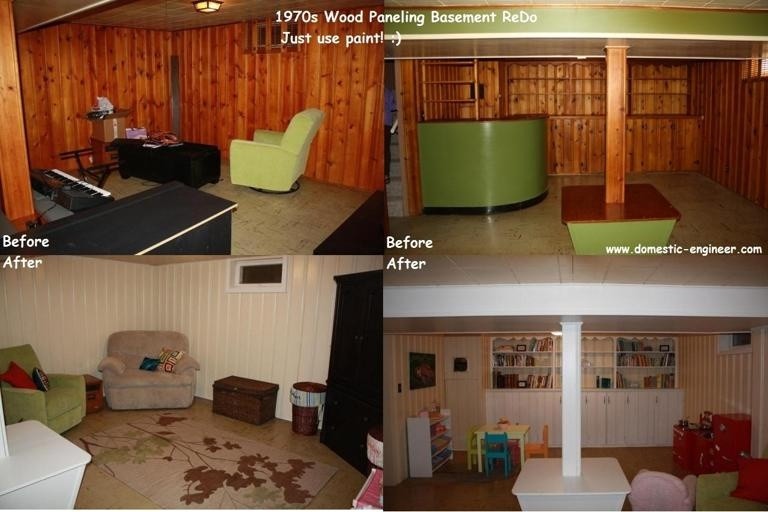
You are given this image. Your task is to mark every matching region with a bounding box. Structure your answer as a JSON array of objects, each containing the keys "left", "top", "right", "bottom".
[
  {"left": 1, "top": 360, "right": 49, "bottom": 392},
  {"left": 729, "top": 454, "right": 767, "bottom": 502},
  {"left": 139, "top": 345, "right": 186, "bottom": 373}
]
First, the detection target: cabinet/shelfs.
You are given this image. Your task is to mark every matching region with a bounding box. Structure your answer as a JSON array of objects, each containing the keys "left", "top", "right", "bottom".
[
  {"left": 672, "top": 411, "right": 752, "bottom": 477},
  {"left": 487, "top": 335, "right": 681, "bottom": 447},
  {"left": 407, "top": 408, "right": 454, "bottom": 479},
  {"left": 81, "top": 373, "right": 104, "bottom": 414}
]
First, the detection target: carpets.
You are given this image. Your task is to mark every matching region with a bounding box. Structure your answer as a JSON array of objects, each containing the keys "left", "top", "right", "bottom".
[{"left": 70, "top": 411, "right": 339, "bottom": 509}]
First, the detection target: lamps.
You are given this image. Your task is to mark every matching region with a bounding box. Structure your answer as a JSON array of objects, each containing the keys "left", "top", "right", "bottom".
[{"left": 191, "top": 0, "right": 225, "bottom": 14}]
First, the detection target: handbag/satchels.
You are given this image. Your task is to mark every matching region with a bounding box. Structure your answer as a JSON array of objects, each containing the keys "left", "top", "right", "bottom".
[
  {"left": 145, "top": 130, "right": 182, "bottom": 146},
  {"left": 126, "top": 119, "right": 147, "bottom": 139}
]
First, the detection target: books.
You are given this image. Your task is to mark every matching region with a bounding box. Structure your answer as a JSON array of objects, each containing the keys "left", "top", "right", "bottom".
[
  {"left": 616, "top": 371, "right": 674, "bottom": 388},
  {"left": 493, "top": 354, "right": 533, "bottom": 367},
  {"left": 632, "top": 342, "right": 643, "bottom": 352},
  {"left": 617, "top": 351, "right": 675, "bottom": 366},
  {"left": 527, "top": 338, "right": 553, "bottom": 351},
  {"left": 493, "top": 371, "right": 552, "bottom": 387}
]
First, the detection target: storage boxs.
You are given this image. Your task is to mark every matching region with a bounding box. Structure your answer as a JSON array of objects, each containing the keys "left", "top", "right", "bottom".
[
  {"left": 92, "top": 117, "right": 127, "bottom": 166},
  {"left": 212, "top": 375, "right": 279, "bottom": 426}
]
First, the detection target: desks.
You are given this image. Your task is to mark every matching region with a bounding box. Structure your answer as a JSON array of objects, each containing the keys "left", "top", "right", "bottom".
[
  {"left": 1, "top": 179, "right": 237, "bottom": 256},
  {"left": 112, "top": 138, "right": 220, "bottom": 188}
]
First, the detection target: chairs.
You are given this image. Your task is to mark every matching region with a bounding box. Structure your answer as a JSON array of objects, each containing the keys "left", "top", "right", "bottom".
[
  {"left": 696, "top": 472, "right": 768, "bottom": 511},
  {"left": 0, "top": 343, "right": 86, "bottom": 437},
  {"left": 466, "top": 417, "right": 549, "bottom": 479},
  {"left": 97, "top": 330, "right": 200, "bottom": 411},
  {"left": 628, "top": 468, "right": 697, "bottom": 511},
  {"left": 228, "top": 108, "right": 324, "bottom": 198}
]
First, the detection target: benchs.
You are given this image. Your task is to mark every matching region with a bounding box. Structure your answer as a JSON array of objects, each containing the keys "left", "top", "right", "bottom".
[
  {"left": 561, "top": 184, "right": 681, "bottom": 255},
  {"left": 0, "top": 419, "right": 91, "bottom": 510},
  {"left": 511, "top": 457, "right": 632, "bottom": 511}
]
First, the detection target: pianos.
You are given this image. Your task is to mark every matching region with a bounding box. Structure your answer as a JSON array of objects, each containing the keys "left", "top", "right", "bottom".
[{"left": 28, "top": 167, "right": 115, "bottom": 214}]
